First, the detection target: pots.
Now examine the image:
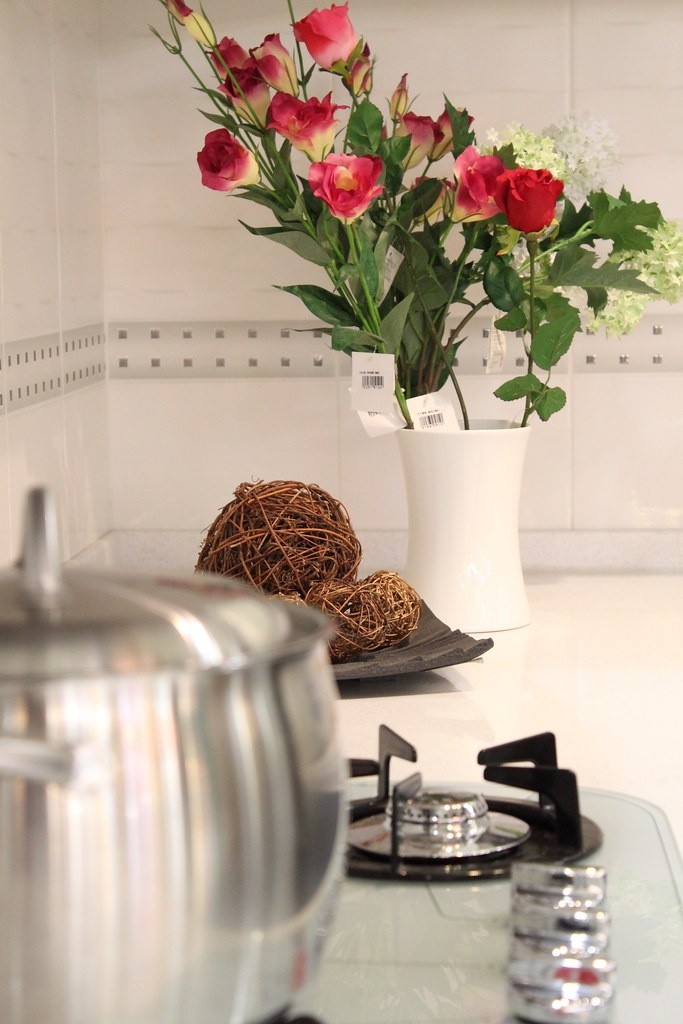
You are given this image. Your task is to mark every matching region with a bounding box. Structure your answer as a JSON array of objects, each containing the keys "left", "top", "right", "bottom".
[{"left": 0, "top": 487, "right": 352, "bottom": 1024}]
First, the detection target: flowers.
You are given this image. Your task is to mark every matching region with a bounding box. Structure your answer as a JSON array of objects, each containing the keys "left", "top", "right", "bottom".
[{"left": 146, "top": 0, "right": 683, "bottom": 430}]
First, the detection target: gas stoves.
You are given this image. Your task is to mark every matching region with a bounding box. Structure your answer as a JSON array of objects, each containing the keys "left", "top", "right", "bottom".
[{"left": 284, "top": 726, "right": 683, "bottom": 1024}]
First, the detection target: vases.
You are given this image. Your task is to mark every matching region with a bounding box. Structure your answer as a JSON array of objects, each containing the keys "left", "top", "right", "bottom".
[{"left": 394, "top": 419, "right": 529, "bottom": 633}]
